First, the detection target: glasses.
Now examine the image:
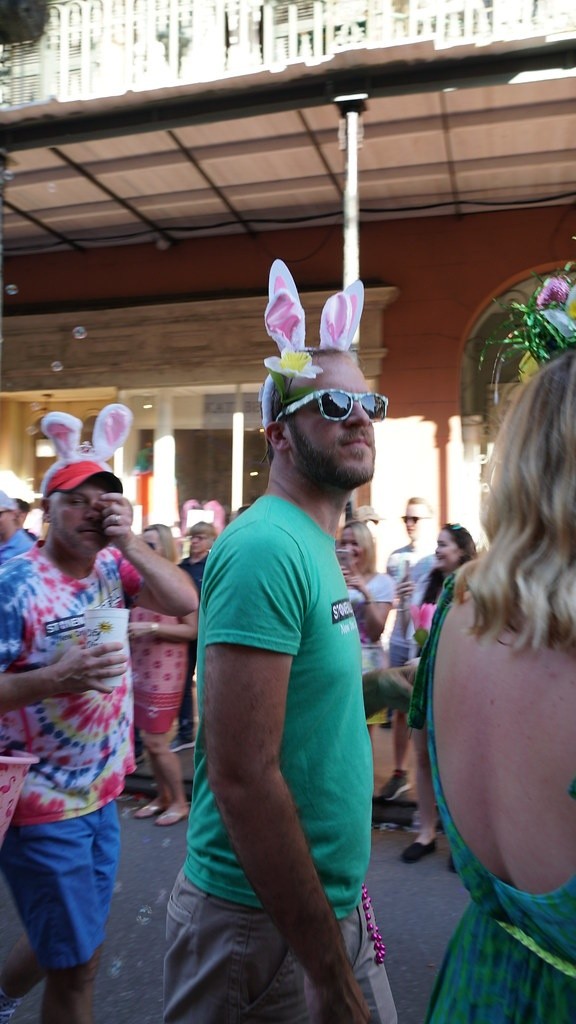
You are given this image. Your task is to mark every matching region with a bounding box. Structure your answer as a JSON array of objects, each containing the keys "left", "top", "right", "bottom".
[
  {"left": 275, "top": 389, "right": 389, "bottom": 425},
  {"left": 401, "top": 516, "right": 429, "bottom": 525},
  {"left": 147, "top": 542, "right": 164, "bottom": 550},
  {"left": 189, "top": 535, "right": 210, "bottom": 542}
]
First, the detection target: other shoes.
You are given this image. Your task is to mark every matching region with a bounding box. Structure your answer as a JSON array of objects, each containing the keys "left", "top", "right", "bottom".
[{"left": 402, "top": 838, "right": 436, "bottom": 862}]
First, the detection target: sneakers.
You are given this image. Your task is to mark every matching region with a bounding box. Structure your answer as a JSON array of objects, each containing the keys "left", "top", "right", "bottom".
[
  {"left": 170, "top": 731, "right": 196, "bottom": 753},
  {"left": 379, "top": 777, "right": 411, "bottom": 802}
]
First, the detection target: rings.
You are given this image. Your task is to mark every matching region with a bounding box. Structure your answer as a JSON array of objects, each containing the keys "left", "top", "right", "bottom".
[{"left": 116, "top": 515, "right": 120, "bottom": 523}]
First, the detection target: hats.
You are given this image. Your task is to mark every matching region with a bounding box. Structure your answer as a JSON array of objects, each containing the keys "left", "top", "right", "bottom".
[
  {"left": 399, "top": 497, "right": 436, "bottom": 519},
  {"left": 0, "top": 470, "right": 34, "bottom": 504},
  {"left": 351, "top": 504, "right": 382, "bottom": 525},
  {"left": 45, "top": 462, "right": 124, "bottom": 497}
]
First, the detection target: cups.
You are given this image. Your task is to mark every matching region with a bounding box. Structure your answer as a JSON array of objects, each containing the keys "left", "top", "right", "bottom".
[
  {"left": 83, "top": 606, "right": 129, "bottom": 686},
  {"left": 0, "top": 749, "right": 39, "bottom": 851}
]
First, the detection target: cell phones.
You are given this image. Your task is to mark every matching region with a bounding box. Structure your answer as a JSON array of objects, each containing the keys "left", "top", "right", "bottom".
[
  {"left": 335, "top": 549, "right": 354, "bottom": 575},
  {"left": 403, "top": 561, "right": 410, "bottom": 585}
]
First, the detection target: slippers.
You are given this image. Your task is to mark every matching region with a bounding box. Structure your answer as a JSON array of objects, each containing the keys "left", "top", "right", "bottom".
[
  {"left": 136, "top": 803, "right": 168, "bottom": 819},
  {"left": 157, "top": 805, "right": 188, "bottom": 826}
]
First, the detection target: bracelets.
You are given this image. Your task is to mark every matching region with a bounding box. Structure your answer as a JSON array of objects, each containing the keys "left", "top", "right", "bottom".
[{"left": 151, "top": 622, "right": 159, "bottom": 636}]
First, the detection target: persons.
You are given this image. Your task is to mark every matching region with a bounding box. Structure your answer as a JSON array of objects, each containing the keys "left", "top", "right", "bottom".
[
  {"left": 0, "top": 461, "right": 199, "bottom": 1024},
  {"left": 0, "top": 471, "right": 38, "bottom": 565},
  {"left": 408, "top": 348, "right": 576, "bottom": 1024},
  {"left": 335, "top": 498, "right": 474, "bottom": 874},
  {"left": 164, "top": 349, "right": 400, "bottom": 1024},
  {"left": 126, "top": 522, "right": 214, "bottom": 826}
]
